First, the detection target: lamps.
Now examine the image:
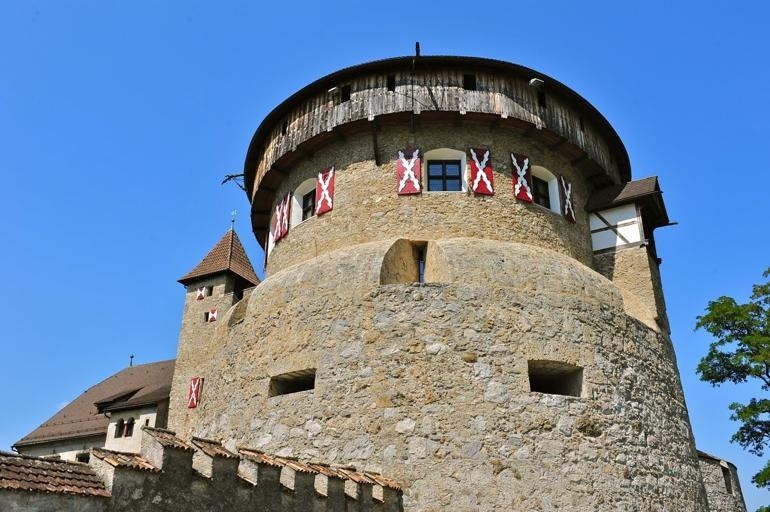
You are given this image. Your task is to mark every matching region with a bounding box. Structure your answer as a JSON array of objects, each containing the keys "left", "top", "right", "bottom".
[
  {"left": 328, "top": 87, "right": 339, "bottom": 94},
  {"left": 529, "top": 78, "right": 543, "bottom": 87}
]
